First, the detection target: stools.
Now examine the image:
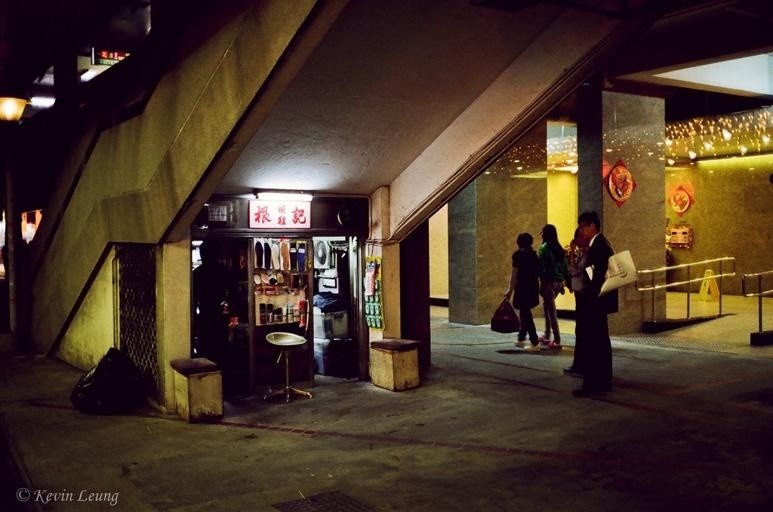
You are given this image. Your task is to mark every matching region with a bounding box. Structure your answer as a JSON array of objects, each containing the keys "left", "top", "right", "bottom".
[
  {"left": 260, "top": 332, "right": 313, "bottom": 403},
  {"left": 367, "top": 338, "right": 422, "bottom": 392},
  {"left": 170, "top": 356, "right": 224, "bottom": 423}
]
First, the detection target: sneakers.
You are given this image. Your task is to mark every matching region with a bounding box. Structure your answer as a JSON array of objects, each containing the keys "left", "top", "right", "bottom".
[
  {"left": 563, "top": 363, "right": 614, "bottom": 396},
  {"left": 516, "top": 336, "right": 563, "bottom": 352}
]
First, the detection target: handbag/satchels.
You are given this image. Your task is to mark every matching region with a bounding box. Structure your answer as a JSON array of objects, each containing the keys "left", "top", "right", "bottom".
[
  {"left": 488, "top": 297, "right": 522, "bottom": 333},
  {"left": 583, "top": 239, "right": 641, "bottom": 298}
]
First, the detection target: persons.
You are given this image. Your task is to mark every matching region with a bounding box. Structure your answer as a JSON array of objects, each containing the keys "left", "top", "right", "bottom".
[
  {"left": 567, "top": 209, "right": 619, "bottom": 397},
  {"left": 502, "top": 232, "right": 542, "bottom": 353},
  {"left": 561, "top": 226, "right": 590, "bottom": 380},
  {"left": 188, "top": 240, "right": 237, "bottom": 397},
  {"left": 536, "top": 224, "right": 574, "bottom": 350}
]
